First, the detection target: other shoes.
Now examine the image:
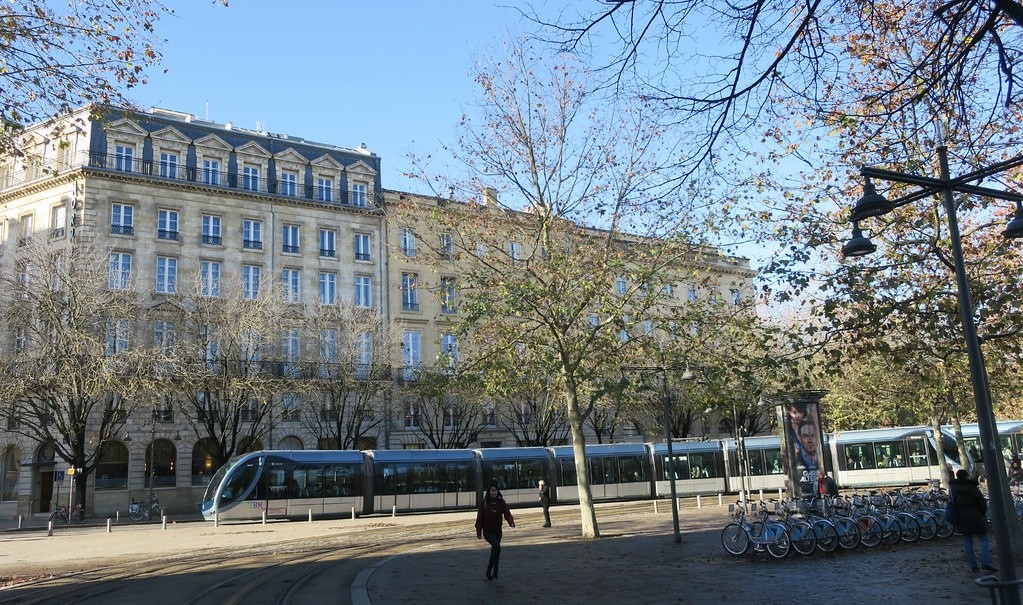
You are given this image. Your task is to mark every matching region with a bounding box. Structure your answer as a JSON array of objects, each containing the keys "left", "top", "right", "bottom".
[
  {"left": 972, "top": 567, "right": 980, "bottom": 572},
  {"left": 981, "top": 563, "right": 999, "bottom": 573},
  {"left": 543, "top": 524, "right": 551, "bottom": 528}
]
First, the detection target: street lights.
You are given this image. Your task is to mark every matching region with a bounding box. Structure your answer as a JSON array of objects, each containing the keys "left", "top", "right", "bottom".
[
  {"left": 701, "top": 394, "right": 766, "bottom": 514},
  {"left": 841, "top": 163, "right": 1023, "bottom": 605},
  {"left": 124, "top": 429, "right": 183, "bottom": 520},
  {"left": 616, "top": 362, "right": 712, "bottom": 544}
]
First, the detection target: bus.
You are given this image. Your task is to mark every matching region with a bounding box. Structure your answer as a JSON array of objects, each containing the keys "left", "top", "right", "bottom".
[
  {"left": 943, "top": 419, "right": 1022, "bottom": 483},
  {"left": 201, "top": 424, "right": 983, "bottom": 520}
]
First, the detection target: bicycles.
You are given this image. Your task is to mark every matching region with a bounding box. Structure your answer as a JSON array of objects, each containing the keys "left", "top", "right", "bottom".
[
  {"left": 48, "top": 502, "right": 89, "bottom": 524},
  {"left": 721, "top": 472, "right": 1023, "bottom": 560},
  {"left": 129, "top": 494, "right": 161, "bottom": 522}
]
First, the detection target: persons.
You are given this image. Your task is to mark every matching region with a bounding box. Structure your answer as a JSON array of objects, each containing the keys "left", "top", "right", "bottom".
[
  {"left": 475, "top": 485, "right": 515, "bottom": 581},
  {"left": 881, "top": 453, "right": 888, "bottom": 467},
  {"left": 538, "top": 480, "right": 552, "bottom": 527},
  {"left": 691, "top": 463, "right": 701, "bottom": 479},
  {"left": 61, "top": 503, "right": 67, "bottom": 523},
  {"left": 825, "top": 471, "right": 839, "bottom": 499},
  {"left": 333, "top": 483, "right": 349, "bottom": 496},
  {"left": 311, "top": 484, "right": 322, "bottom": 497},
  {"left": 634, "top": 471, "right": 642, "bottom": 481},
  {"left": 893, "top": 452, "right": 902, "bottom": 463},
  {"left": 1011, "top": 452, "right": 1021, "bottom": 487},
  {"left": 861, "top": 455, "right": 873, "bottom": 468},
  {"left": 944, "top": 469, "right": 999, "bottom": 573},
  {"left": 847, "top": 455, "right": 854, "bottom": 469},
  {"left": 817, "top": 470, "right": 828, "bottom": 494},
  {"left": 789, "top": 404, "right": 819, "bottom": 470}
]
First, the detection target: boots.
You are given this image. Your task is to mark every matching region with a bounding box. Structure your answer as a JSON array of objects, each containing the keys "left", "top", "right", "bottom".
[
  {"left": 485, "top": 568, "right": 493, "bottom": 580},
  {"left": 493, "top": 567, "right": 498, "bottom": 578}
]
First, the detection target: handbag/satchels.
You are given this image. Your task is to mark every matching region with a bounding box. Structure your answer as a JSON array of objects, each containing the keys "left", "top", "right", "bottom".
[{"left": 943, "top": 487, "right": 957, "bottom": 525}]
